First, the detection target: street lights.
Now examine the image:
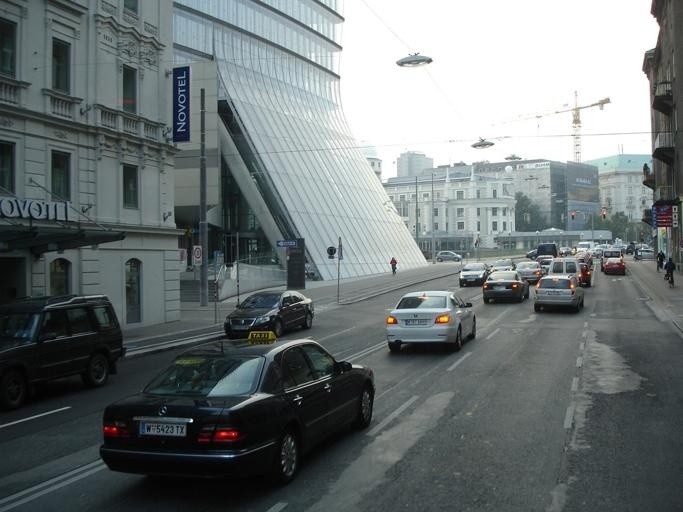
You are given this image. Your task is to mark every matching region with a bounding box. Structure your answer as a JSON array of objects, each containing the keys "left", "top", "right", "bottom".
[
  {"left": 535, "top": 230, "right": 539, "bottom": 249},
  {"left": 576, "top": 211, "right": 586, "bottom": 230},
  {"left": 558, "top": 232, "right": 563, "bottom": 247},
  {"left": 580, "top": 233, "right": 584, "bottom": 242},
  {"left": 476, "top": 232, "right": 481, "bottom": 262},
  {"left": 599, "top": 234, "right": 602, "bottom": 244},
  {"left": 507, "top": 231, "right": 512, "bottom": 258}
]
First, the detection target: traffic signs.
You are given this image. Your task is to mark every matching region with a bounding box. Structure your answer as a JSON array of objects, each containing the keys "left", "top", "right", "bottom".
[
  {"left": 652, "top": 220, "right": 678, "bottom": 227},
  {"left": 276, "top": 240, "right": 298, "bottom": 248},
  {"left": 650, "top": 206, "right": 677, "bottom": 213},
  {"left": 651, "top": 214, "right": 676, "bottom": 220}
]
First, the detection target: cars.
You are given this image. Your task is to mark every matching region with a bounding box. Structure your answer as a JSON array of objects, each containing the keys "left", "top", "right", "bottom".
[
  {"left": 223, "top": 290, "right": 312, "bottom": 341},
  {"left": 98, "top": 331, "right": 375, "bottom": 490},
  {"left": 435, "top": 236, "right": 656, "bottom": 312},
  {"left": 384, "top": 290, "right": 476, "bottom": 352}
]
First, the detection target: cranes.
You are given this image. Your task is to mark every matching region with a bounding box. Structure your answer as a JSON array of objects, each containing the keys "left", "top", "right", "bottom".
[{"left": 536, "top": 89, "right": 610, "bottom": 166}]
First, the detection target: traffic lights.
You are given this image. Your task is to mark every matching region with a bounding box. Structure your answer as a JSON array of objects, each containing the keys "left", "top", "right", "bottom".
[
  {"left": 570, "top": 210, "right": 575, "bottom": 220},
  {"left": 601, "top": 209, "right": 606, "bottom": 219}
]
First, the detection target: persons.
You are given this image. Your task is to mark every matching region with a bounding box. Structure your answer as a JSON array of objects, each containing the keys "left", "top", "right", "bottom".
[
  {"left": 663, "top": 257, "right": 675, "bottom": 280},
  {"left": 654, "top": 249, "right": 665, "bottom": 273},
  {"left": 389, "top": 257, "right": 397, "bottom": 277}
]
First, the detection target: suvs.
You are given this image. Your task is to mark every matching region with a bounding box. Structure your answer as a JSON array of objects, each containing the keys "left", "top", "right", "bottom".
[{"left": 0, "top": 290, "right": 129, "bottom": 410}]
